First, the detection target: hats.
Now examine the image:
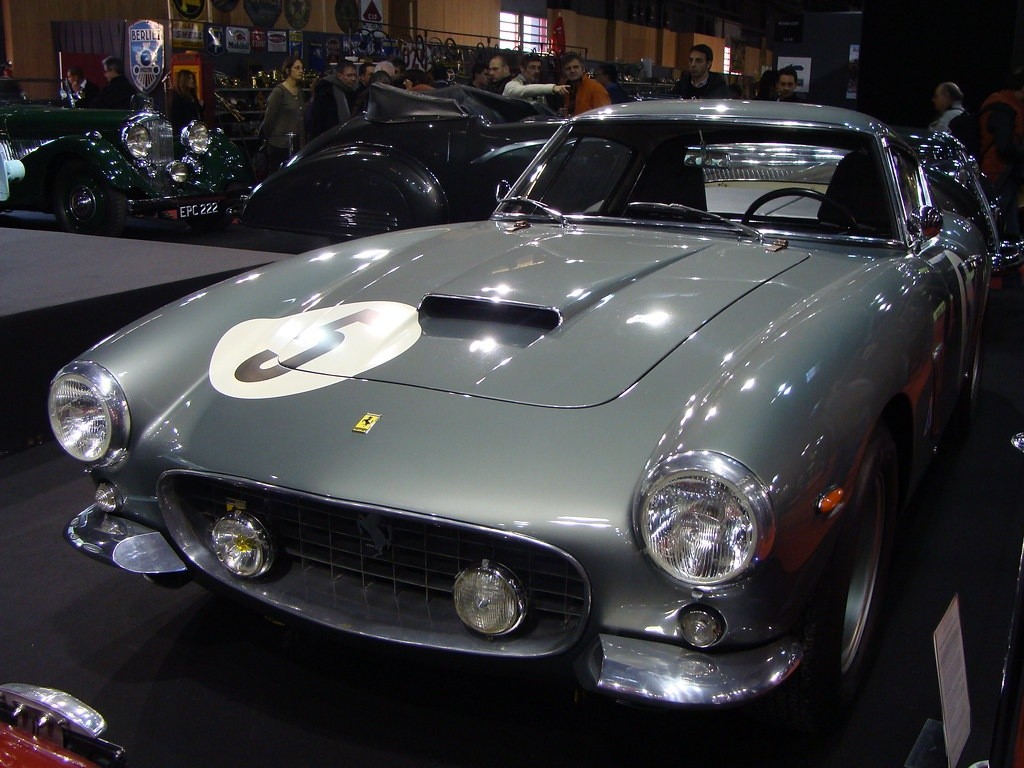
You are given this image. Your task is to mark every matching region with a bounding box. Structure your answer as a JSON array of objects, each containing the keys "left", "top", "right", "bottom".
[{"left": 372, "top": 60, "right": 395, "bottom": 78}]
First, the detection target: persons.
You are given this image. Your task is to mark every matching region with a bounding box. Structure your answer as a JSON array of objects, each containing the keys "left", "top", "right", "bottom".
[
  {"left": 932, "top": 67, "right": 1024, "bottom": 288},
  {"left": 679, "top": 44, "right": 727, "bottom": 99},
  {"left": 469, "top": 53, "right": 627, "bottom": 118},
  {"left": 160, "top": 70, "right": 202, "bottom": 131},
  {"left": 257, "top": 58, "right": 443, "bottom": 173},
  {"left": 90, "top": 56, "right": 137, "bottom": 110},
  {"left": 726, "top": 70, "right": 803, "bottom": 103},
  {"left": 67, "top": 68, "right": 99, "bottom": 109}
]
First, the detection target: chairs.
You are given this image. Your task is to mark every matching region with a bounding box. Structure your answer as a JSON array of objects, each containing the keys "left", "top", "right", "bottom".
[
  {"left": 619, "top": 161, "right": 707, "bottom": 224},
  {"left": 816, "top": 152, "right": 904, "bottom": 239}
]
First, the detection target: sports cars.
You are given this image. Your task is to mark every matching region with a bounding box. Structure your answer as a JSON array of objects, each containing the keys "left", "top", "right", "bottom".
[
  {"left": 0, "top": 62, "right": 661, "bottom": 245},
  {"left": 43, "top": 95, "right": 1024, "bottom": 756}
]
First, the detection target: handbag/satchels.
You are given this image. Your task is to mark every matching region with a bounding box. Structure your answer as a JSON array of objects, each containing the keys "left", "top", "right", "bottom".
[{"left": 968, "top": 161, "right": 1020, "bottom": 241}]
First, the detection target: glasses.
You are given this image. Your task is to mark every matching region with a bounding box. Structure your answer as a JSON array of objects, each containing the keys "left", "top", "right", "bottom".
[{"left": 488, "top": 67, "right": 499, "bottom": 72}]
